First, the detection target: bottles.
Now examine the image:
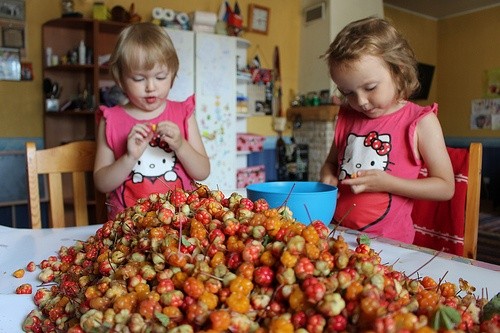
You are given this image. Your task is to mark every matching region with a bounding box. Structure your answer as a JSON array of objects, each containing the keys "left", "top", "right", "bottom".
[
  {"left": 45, "top": 38, "right": 93, "bottom": 68},
  {"left": 93, "top": 1, "right": 107, "bottom": 21},
  {"left": 292, "top": 89, "right": 331, "bottom": 106}
]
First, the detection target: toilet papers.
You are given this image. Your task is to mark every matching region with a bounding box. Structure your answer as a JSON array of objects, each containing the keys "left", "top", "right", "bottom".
[
  {"left": 176, "top": 12, "right": 189, "bottom": 24},
  {"left": 192, "top": 25, "right": 215, "bottom": 34},
  {"left": 163, "top": 9, "right": 175, "bottom": 21},
  {"left": 190, "top": 10, "right": 217, "bottom": 26},
  {"left": 152, "top": 7, "right": 163, "bottom": 19}
]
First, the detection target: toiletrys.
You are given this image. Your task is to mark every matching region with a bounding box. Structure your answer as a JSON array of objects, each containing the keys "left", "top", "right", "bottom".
[{"left": 78, "top": 39, "right": 87, "bottom": 65}]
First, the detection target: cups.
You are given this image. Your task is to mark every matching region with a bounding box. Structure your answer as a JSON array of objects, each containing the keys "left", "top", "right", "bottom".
[{"left": 46, "top": 98, "right": 60, "bottom": 111}]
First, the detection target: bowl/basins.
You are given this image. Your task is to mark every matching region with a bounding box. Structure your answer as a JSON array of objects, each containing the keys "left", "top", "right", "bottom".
[{"left": 246, "top": 181, "right": 339, "bottom": 230}]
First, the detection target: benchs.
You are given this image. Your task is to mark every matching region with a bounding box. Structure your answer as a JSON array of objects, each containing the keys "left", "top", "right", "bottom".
[{"left": 446, "top": 136, "right": 500, "bottom": 264}]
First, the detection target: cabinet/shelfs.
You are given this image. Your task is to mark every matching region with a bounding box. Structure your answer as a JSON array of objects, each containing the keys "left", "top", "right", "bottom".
[
  {"left": 160, "top": 28, "right": 253, "bottom": 202},
  {"left": 41, "top": 17, "right": 129, "bottom": 229},
  {"left": 0, "top": 0, "right": 27, "bottom": 52}
]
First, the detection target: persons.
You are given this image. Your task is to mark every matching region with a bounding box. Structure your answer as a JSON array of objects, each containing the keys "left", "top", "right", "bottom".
[
  {"left": 93, "top": 23, "right": 211, "bottom": 222},
  {"left": 320, "top": 18, "right": 455, "bottom": 245}
]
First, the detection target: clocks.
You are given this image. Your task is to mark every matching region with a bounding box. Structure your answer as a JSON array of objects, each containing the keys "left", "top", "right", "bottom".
[{"left": 248, "top": 4, "right": 271, "bottom": 35}]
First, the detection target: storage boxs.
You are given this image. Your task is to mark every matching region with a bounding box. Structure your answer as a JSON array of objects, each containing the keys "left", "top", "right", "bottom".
[
  {"left": 237, "top": 133, "right": 265, "bottom": 151},
  {"left": 238, "top": 166, "right": 265, "bottom": 188}
]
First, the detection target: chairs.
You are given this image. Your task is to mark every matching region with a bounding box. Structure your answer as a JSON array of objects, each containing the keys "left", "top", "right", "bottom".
[
  {"left": 410, "top": 142, "right": 483, "bottom": 260},
  {"left": 25, "top": 140, "right": 107, "bottom": 229}
]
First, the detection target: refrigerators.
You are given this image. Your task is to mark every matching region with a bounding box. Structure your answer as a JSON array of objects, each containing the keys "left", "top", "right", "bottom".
[{"left": 157, "top": 26, "right": 238, "bottom": 200}]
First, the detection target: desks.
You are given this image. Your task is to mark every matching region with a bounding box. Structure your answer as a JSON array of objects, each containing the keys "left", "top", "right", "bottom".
[{"left": 0, "top": 221, "right": 500, "bottom": 333}]
